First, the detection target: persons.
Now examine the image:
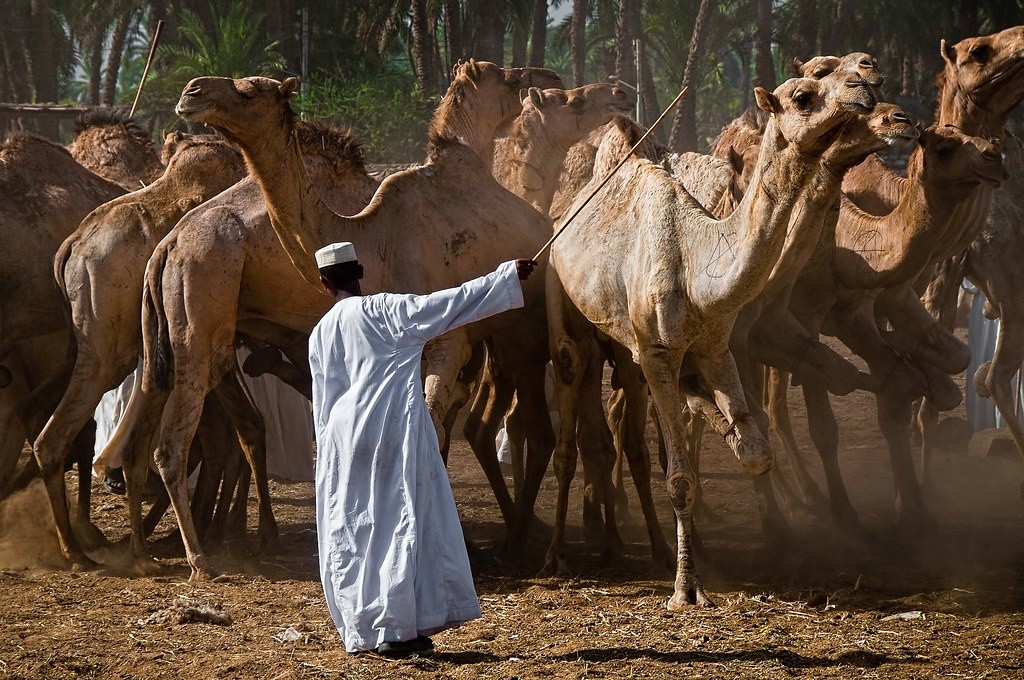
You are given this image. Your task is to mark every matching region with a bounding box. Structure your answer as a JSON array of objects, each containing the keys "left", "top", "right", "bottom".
[
  {"left": 309, "top": 242, "right": 538, "bottom": 656},
  {"left": 93, "top": 341, "right": 314, "bottom": 495},
  {"left": 494, "top": 423, "right": 529, "bottom": 473},
  {"left": 963, "top": 277, "right": 1024, "bottom": 433}
]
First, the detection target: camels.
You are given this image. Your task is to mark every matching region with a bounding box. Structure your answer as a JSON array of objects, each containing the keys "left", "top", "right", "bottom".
[{"left": 0, "top": 25, "right": 1024, "bottom": 614}]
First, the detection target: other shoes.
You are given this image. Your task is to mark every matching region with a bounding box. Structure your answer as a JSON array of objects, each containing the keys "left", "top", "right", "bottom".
[{"left": 379, "top": 635, "right": 434, "bottom": 657}]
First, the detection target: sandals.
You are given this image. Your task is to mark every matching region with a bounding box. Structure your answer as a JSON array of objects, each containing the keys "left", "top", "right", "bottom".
[{"left": 102, "top": 467, "right": 126, "bottom": 495}]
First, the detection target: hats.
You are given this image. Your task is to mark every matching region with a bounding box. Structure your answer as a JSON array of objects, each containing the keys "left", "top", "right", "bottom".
[{"left": 314, "top": 242, "right": 357, "bottom": 269}]
